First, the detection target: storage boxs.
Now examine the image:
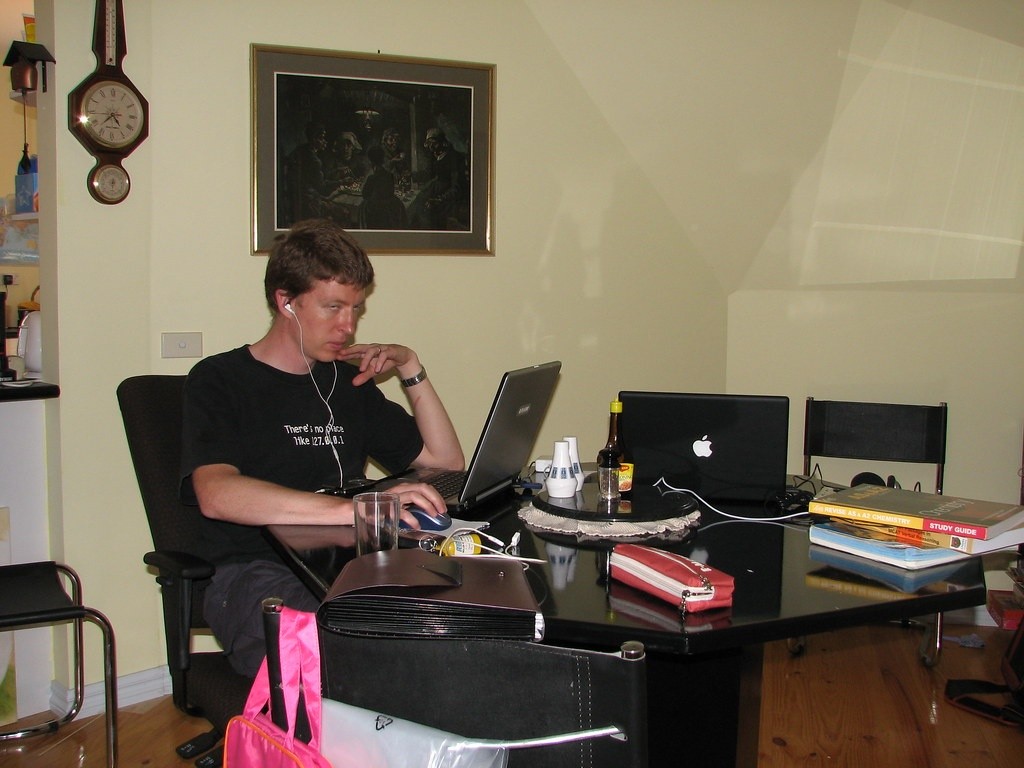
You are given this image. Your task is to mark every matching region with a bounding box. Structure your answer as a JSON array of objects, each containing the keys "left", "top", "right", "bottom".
[{"left": 14, "top": 172, "right": 38, "bottom": 213}]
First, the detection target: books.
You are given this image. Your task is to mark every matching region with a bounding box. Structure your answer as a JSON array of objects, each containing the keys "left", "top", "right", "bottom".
[{"left": 807, "top": 484, "right": 1024, "bottom": 570}]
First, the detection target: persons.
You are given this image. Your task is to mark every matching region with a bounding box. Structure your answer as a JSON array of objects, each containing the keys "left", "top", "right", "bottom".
[{"left": 174, "top": 220, "right": 466, "bottom": 694}]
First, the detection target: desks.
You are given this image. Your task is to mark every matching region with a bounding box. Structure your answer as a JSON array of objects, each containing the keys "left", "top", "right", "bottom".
[{"left": 263, "top": 463, "right": 992, "bottom": 768}]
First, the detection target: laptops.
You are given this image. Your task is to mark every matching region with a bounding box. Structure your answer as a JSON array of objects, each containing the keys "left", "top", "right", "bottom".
[
  {"left": 616, "top": 391, "right": 789, "bottom": 502},
  {"left": 340, "top": 360, "right": 562, "bottom": 511}
]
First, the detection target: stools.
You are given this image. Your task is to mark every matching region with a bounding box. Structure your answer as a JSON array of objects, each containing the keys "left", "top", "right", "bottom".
[{"left": 0, "top": 556, "right": 121, "bottom": 768}]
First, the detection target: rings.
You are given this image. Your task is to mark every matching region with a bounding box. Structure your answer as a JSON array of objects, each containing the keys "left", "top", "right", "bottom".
[{"left": 377, "top": 346, "right": 381, "bottom": 353}]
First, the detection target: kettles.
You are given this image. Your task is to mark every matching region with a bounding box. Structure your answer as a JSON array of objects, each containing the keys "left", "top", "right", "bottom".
[{"left": 17, "top": 311, "right": 41, "bottom": 378}]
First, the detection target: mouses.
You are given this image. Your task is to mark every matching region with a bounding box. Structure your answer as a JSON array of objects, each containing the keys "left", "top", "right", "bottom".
[{"left": 383, "top": 501, "right": 452, "bottom": 531}]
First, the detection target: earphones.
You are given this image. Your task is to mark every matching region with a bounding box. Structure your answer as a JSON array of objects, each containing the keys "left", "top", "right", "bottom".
[{"left": 284, "top": 301, "right": 296, "bottom": 314}]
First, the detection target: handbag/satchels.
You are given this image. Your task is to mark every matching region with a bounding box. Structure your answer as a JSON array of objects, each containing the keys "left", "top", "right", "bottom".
[
  {"left": 315, "top": 551, "right": 546, "bottom": 643},
  {"left": 222, "top": 611, "right": 334, "bottom": 768}
]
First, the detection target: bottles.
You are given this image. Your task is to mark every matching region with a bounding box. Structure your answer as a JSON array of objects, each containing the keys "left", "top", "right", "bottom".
[
  {"left": 598, "top": 449, "right": 621, "bottom": 499},
  {"left": 545, "top": 442, "right": 578, "bottom": 497},
  {"left": 605, "top": 401, "right": 634, "bottom": 499},
  {"left": 617, "top": 500, "right": 633, "bottom": 515},
  {"left": 562, "top": 436, "right": 586, "bottom": 492}
]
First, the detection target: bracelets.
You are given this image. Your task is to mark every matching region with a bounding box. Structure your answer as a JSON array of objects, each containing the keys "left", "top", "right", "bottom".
[{"left": 401, "top": 367, "right": 427, "bottom": 387}]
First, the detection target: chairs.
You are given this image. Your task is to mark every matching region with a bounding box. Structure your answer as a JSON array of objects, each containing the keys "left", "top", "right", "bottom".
[
  {"left": 798, "top": 393, "right": 949, "bottom": 671},
  {"left": 114, "top": 365, "right": 283, "bottom": 768},
  {"left": 256, "top": 596, "right": 696, "bottom": 768}
]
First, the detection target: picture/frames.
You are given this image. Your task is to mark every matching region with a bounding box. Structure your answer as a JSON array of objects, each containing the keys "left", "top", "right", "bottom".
[{"left": 247, "top": 40, "right": 503, "bottom": 263}]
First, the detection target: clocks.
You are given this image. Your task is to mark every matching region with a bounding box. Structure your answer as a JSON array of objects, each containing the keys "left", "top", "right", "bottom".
[{"left": 67, "top": 0, "right": 150, "bottom": 205}]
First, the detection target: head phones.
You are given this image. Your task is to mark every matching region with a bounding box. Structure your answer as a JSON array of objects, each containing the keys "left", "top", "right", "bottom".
[{"left": 851, "top": 472, "right": 901, "bottom": 490}]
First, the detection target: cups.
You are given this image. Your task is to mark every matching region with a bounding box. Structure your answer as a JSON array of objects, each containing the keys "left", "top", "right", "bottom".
[{"left": 353, "top": 492, "right": 400, "bottom": 556}]
